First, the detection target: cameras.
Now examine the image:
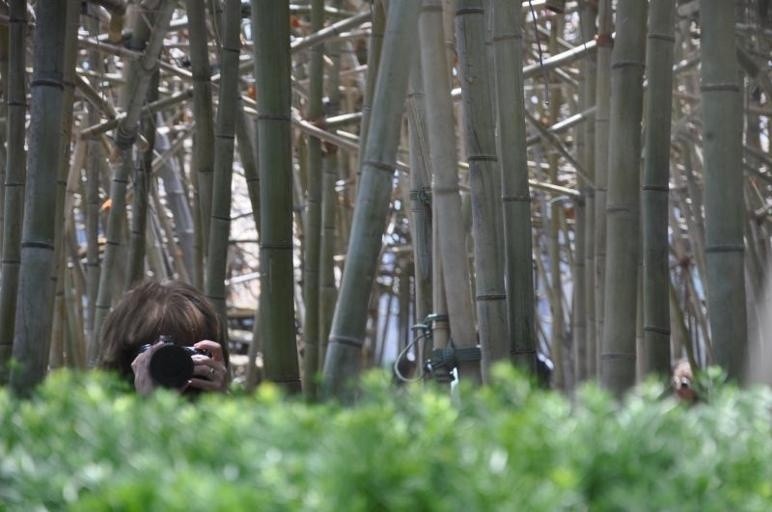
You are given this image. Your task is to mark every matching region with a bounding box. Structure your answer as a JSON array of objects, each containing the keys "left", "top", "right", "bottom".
[{"left": 140, "top": 334, "right": 212, "bottom": 392}]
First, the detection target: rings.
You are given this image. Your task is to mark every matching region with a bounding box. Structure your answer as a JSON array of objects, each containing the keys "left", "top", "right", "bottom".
[{"left": 206, "top": 367, "right": 217, "bottom": 380}]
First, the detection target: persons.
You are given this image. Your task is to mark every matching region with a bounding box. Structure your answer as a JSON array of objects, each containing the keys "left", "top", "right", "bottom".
[
  {"left": 445, "top": 321, "right": 557, "bottom": 401},
  {"left": 89, "top": 274, "right": 231, "bottom": 397},
  {"left": 670, "top": 360, "right": 707, "bottom": 407}
]
